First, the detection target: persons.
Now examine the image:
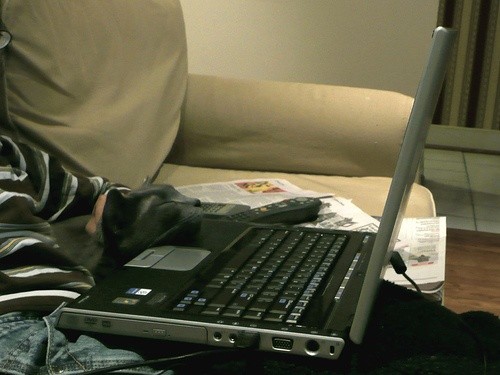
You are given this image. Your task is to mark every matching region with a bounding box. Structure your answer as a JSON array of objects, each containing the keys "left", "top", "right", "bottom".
[{"left": 0, "top": 126, "right": 176, "bottom": 375}]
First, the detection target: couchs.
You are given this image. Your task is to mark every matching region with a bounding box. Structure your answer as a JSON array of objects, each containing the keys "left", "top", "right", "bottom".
[{"left": 0, "top": 0, "right": 436, "bottom": 218}]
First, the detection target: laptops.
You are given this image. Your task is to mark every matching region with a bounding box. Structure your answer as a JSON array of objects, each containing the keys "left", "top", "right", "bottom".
[{"left": 55, "top": 25, "right": 454, "bottom": 359}]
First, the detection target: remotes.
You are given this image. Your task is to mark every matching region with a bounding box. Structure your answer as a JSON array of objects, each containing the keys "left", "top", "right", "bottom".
[
  {"left": 200, "top": 202, "right": 250, "bottom": 219},
  {"left": 227, "top": 196, "right": 323, "bottom": 224}
]
